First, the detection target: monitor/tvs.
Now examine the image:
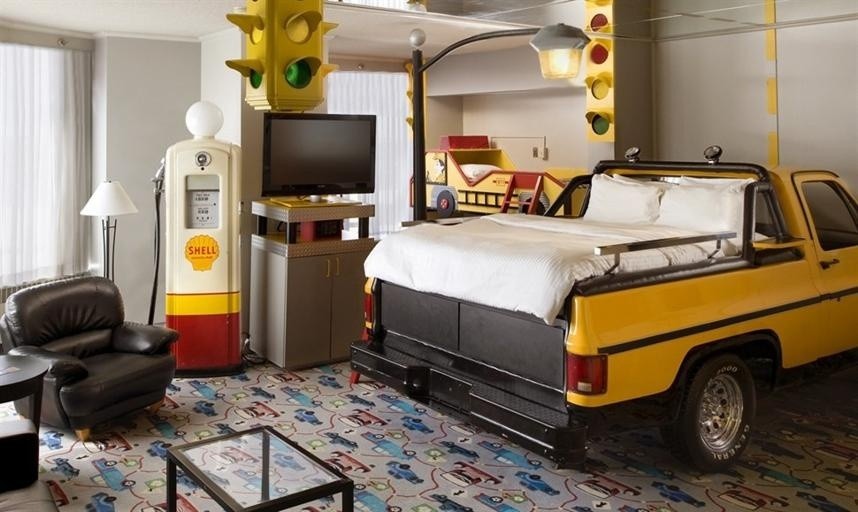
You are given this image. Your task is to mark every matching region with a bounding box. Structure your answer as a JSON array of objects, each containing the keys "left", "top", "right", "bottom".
[{"left": 262, "top": 112, "right": 376, "bottom": 208}]
